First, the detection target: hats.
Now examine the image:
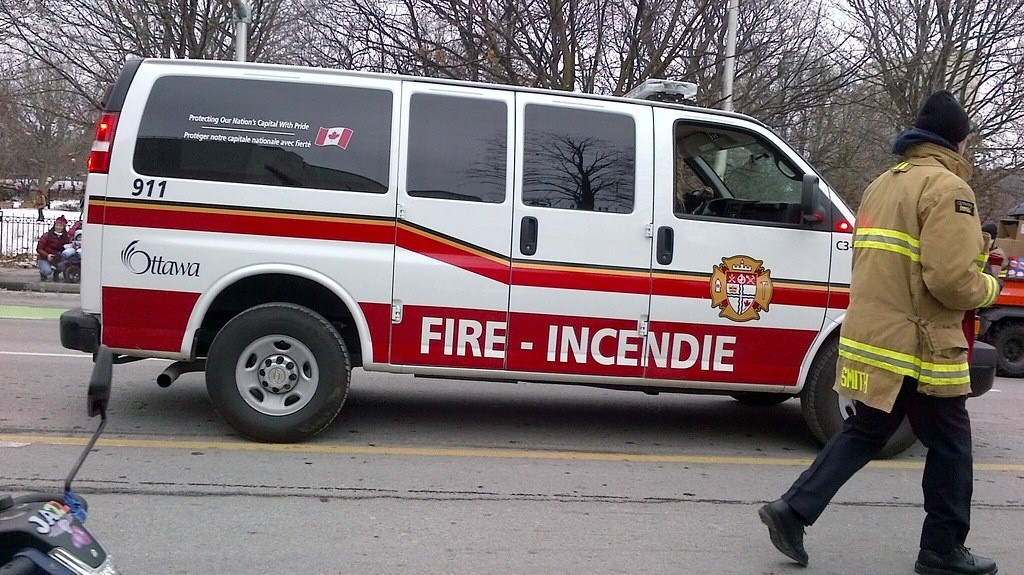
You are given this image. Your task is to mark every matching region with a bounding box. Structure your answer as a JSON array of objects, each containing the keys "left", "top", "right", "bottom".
[
  {"left": 915, "top": 90, "right": 970, "bottom": 141},
  {"left": 56, "top": 215, "right": 67, "bottom": 225},
  {"left": 981, "top": 224, "right": 997, "bottom": 239}
]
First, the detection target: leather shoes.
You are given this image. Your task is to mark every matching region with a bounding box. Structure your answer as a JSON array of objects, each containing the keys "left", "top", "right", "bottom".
[
  {"left": 915, "top": 543, "right": 998, "bottom": 575},
  {"left": 759, "top": 500, "right": 809, "bottom": 567}
]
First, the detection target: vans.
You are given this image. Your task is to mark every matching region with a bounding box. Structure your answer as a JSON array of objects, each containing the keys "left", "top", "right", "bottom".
[{"left": 54, "top": 54, "right": 999, "bottom": 458}]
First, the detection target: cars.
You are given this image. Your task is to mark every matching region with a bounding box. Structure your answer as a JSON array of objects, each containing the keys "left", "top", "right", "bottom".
[{"left": 977, "top": 277, "right": 1024, "bottom": 380}]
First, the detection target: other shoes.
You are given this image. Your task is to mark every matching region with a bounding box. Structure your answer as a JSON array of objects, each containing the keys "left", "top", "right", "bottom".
[
  {"left": 40, "top": 273, "right": 47, "bottom": 281},
  {"left": 53, "top": 273, "right": 59, "bottom": 281}
]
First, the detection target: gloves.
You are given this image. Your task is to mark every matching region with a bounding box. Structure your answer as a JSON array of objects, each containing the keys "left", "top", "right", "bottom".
[{"left": 983, "top": 268, "right": 1004, "bottom": 293}]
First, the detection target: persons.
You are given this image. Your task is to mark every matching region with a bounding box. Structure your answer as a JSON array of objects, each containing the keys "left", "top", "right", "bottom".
[
  {"left": 36, "top": 189, "right": 47, "bottom": 221},
  {"left": 677, "top": 176, "right": 714, "bottom": 214},
  {"left": 61, "top": 229, "right": 83, "bottom": 258},
  {"left": 757, "top": 89, "right": 1004, "bottom": 575},
  {"left": 36, "top": 215, "right": 69, "bottom": 282},
  {"left": 79, "top": 195, "right": 85, "bottom": 220}
]
